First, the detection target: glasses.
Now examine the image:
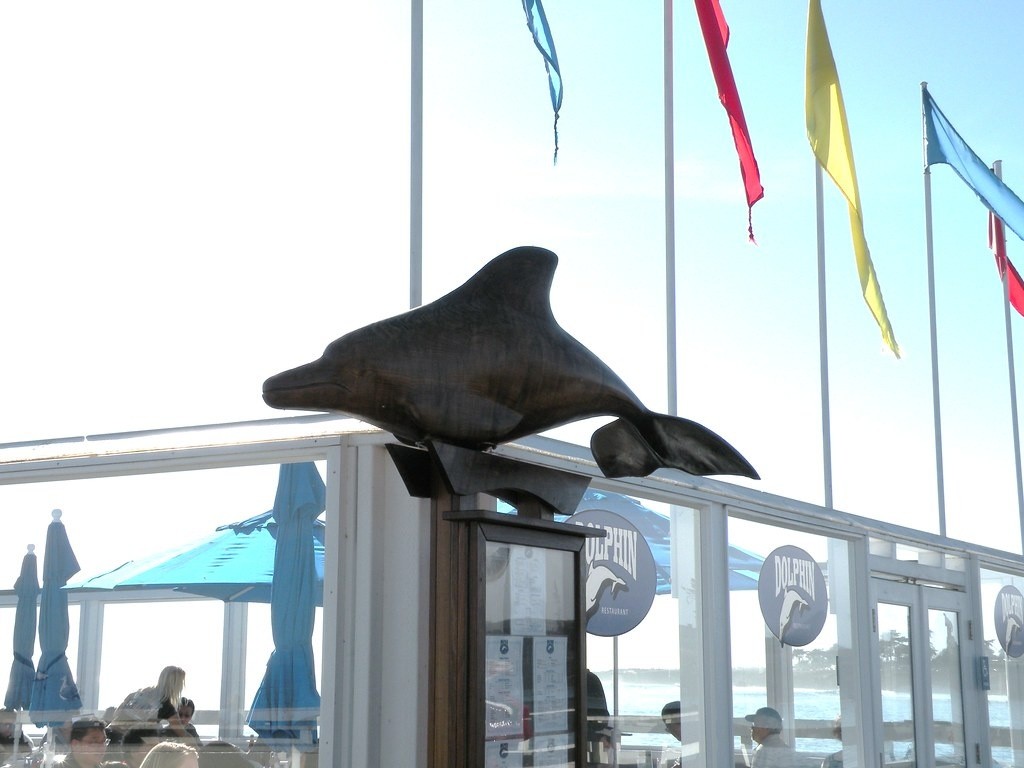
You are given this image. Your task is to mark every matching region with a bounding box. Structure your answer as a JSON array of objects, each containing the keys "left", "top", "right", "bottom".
[
  {"left": 86, "top": 738, "right": 111, "bottom": 747},
  {"left": 665, "top": 723, "right": 670, "bottom": 732},
  {"left": 178, "top": 712, "right": 190, "bottom": 718}
]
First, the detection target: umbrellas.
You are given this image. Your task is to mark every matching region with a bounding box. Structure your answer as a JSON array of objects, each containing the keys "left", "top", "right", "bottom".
[
  {"left": 28, "top": 508, "right": 83, "bottom": 745},
  {"left": 4, "top": 544, "right": 41, "bottom": 760},
  {"left": 111, "top": 462, "right": 326, "bottom": 747},
  {"left": 490, "top": 488, "right": 775, "bottom": 753}
]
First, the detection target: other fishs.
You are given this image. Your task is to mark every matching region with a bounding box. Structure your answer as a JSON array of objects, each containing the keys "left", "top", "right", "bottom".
[{"left": 262, "top": 246, "right": 763, "bottom": 480}]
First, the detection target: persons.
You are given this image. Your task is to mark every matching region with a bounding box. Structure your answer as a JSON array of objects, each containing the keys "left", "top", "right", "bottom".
[
  {"left": 582, "top": 666, "right": 847, "bottom": 768},
  {"left": 0, "top": 664, "right": 259, "bottom": 767}
]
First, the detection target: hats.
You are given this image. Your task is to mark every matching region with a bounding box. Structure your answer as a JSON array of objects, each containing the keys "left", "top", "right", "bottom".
[{"left": 745, "top": 708, "right": 782, "bottom": 725}]
